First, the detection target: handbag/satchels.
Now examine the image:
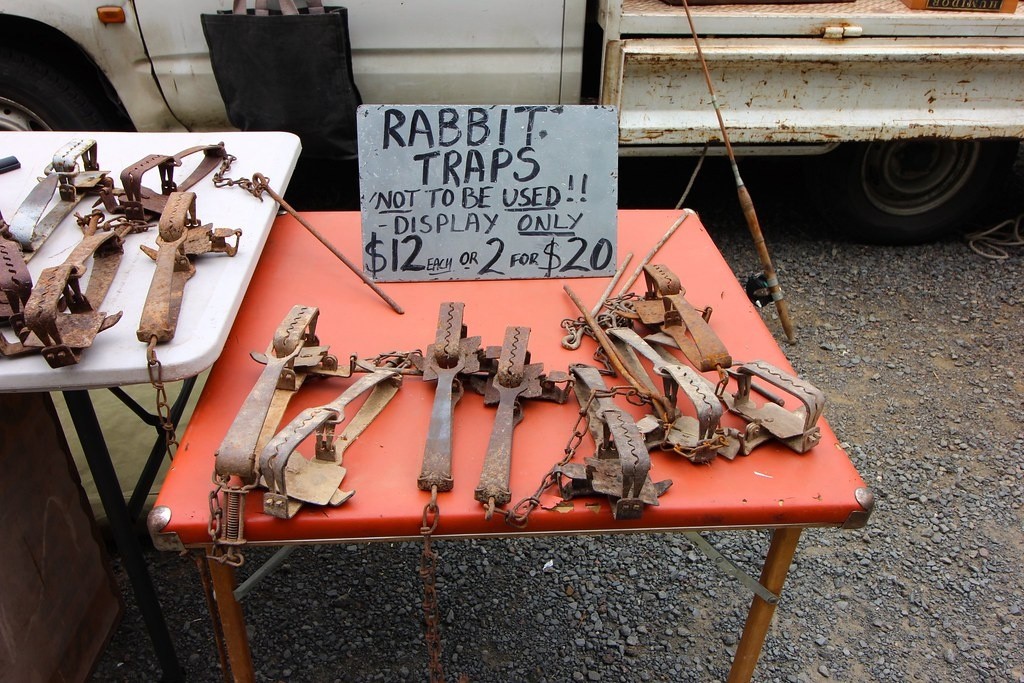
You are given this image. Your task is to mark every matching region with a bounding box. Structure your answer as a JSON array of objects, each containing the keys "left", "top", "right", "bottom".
[{"left": 200, "top": 0, "right": 363, "bottom": 161}]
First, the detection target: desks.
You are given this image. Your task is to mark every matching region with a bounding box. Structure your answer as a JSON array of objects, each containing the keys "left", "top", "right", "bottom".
[
  {"left": 144, "top": 207, "right": 873, "bottom": 683},
  {"left": 0, "top": 130, "right": 303, "bottom": 683}
]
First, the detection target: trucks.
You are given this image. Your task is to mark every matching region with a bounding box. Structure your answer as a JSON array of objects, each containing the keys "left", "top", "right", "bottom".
[{"left": 0, "top": 0, "right": 1024, "bottom": 245}]
